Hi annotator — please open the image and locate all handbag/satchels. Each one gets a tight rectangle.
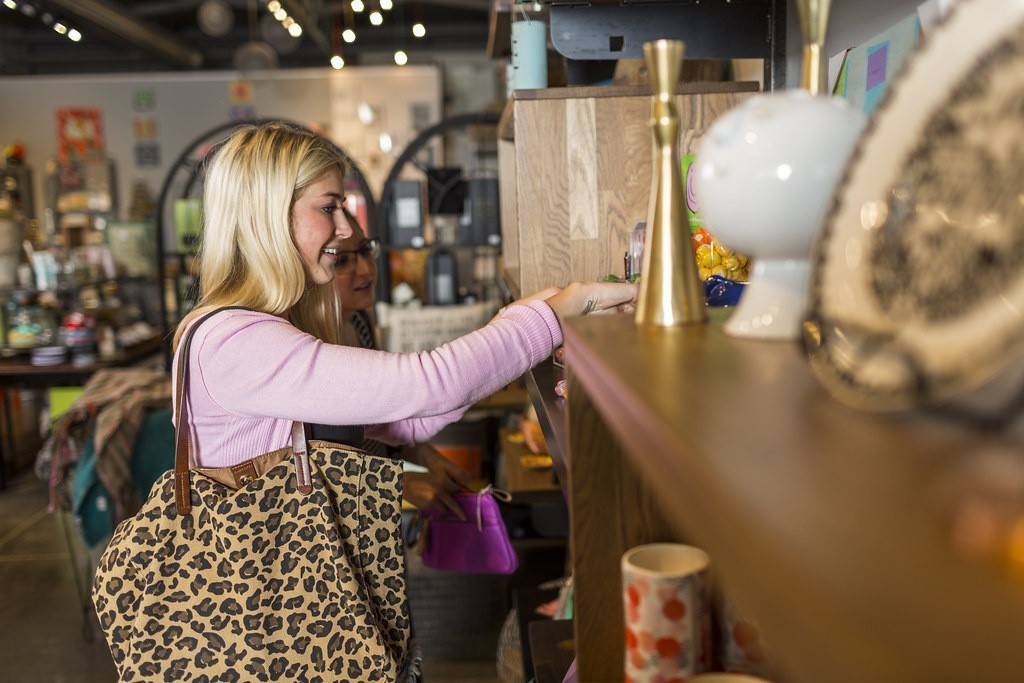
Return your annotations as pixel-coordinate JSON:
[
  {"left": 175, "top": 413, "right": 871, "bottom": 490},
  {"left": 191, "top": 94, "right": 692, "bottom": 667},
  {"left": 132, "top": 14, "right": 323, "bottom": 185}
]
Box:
[
  {"left": 422, "top": 485, "right": 517, "bottom": 574},
  {"left": 92, "top": 305, "right": 413, "bottom": 683}
]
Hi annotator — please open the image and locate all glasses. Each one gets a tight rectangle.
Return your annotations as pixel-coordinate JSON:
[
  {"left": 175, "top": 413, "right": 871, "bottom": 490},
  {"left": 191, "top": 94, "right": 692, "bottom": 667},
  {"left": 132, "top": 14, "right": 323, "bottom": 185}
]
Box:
[{"left": 334, "top": 236, "right": 382, "bottom": 275}]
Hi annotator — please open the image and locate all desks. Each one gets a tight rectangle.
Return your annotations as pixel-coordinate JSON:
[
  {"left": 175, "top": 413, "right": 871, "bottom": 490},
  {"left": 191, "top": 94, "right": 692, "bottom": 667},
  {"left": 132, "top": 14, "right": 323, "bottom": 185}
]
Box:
[{"left": 0, "top": 331, "right": 174, "bottom": 479}]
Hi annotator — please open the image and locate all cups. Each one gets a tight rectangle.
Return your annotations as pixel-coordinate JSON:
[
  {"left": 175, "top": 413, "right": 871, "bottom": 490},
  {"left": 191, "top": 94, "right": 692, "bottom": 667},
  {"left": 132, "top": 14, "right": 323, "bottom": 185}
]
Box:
[{"left": 621, "top": 543, "right": 712, "bottom": 682}]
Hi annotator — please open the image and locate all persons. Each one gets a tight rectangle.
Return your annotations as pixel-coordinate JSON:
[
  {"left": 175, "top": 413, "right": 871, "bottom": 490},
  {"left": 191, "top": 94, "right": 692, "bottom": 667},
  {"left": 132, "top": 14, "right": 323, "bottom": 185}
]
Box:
[{"left": 173, "top": 119, "right": 640, "bottom": 682}]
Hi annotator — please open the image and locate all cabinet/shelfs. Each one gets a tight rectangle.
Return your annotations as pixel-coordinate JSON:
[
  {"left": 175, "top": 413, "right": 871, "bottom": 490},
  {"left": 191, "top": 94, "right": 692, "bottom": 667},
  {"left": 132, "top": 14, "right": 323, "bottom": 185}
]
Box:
[{"left": 496, "top": 81, "right": 1024, "bottom": 683}]
